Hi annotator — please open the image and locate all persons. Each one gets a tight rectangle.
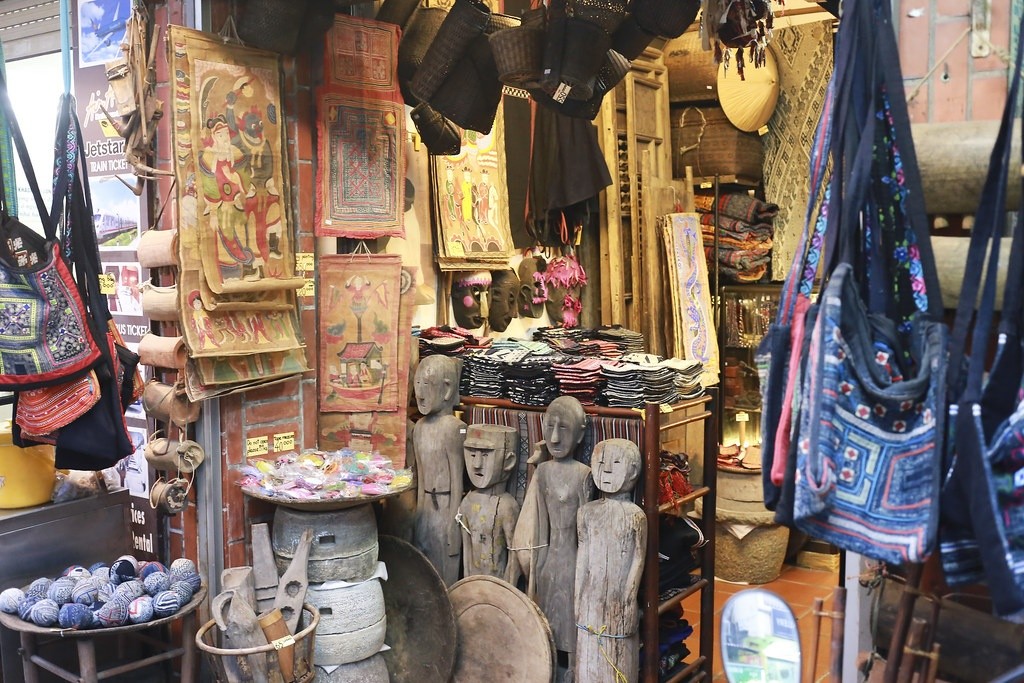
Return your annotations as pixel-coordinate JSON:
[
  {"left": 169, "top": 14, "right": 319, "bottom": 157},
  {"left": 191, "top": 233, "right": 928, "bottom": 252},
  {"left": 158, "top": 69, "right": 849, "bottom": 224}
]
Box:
[
  {"left": 461, "top": 424, "right": 520, "bottom": 585},
  {"left": 413, "top": 353, "right": 467, "bottom": 589},
  {"left": 573, "top": 438, "right": 648, "bottom": 683},
  {"left": 511, "top": 396, "right": 592, "bottom": 683}
]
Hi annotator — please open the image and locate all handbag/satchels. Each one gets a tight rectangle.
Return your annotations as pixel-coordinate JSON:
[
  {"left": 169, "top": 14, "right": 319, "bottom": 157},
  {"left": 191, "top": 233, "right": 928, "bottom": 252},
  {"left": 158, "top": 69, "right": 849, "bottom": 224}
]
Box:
[
  {"left": 751, "top": 1, "right": 1024, "bottom": 614},
  {"left": 0, "top": 74, "right": 139, "bottom": 473}
]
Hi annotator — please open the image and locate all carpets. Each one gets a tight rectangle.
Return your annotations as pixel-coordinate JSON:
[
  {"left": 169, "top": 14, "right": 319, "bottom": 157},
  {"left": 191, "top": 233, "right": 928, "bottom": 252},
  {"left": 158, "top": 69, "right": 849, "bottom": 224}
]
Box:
[{"left": 313, "top": 14, "right": 407, "bottom": 241}]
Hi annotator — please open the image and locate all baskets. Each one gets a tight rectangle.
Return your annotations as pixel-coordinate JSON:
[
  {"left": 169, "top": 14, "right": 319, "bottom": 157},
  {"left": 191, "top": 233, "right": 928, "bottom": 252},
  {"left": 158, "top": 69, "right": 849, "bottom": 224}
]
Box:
[{"left": 373, "top": 0, "right": 699, "bottom": 156}]
